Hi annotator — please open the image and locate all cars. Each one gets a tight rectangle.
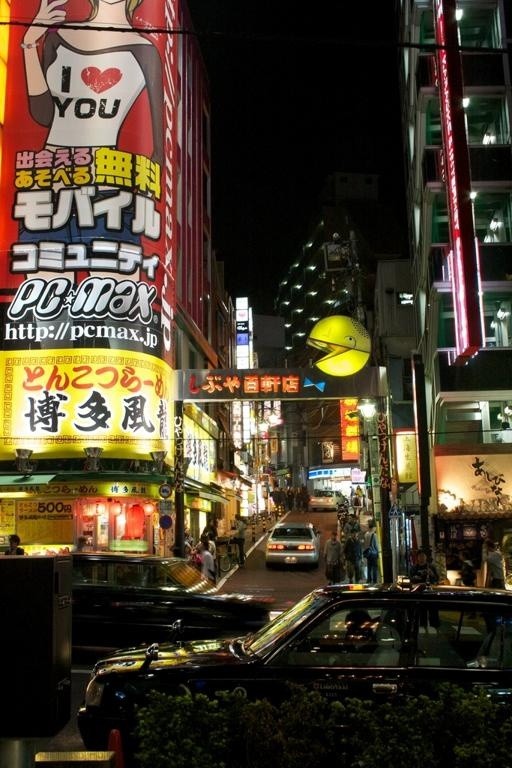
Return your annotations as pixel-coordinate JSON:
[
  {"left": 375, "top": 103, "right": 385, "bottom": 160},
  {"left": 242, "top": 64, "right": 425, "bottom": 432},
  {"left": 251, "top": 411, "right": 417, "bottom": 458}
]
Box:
[{"left": 308, "top": 490, "right": 347, "bottom": 511}]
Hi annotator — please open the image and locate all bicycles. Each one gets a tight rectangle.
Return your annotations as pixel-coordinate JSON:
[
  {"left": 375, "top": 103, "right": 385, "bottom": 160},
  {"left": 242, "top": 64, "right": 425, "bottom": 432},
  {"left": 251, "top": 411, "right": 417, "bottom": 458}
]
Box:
[{"left": 215, "top": 532, "right": 248, "bottom": 572}]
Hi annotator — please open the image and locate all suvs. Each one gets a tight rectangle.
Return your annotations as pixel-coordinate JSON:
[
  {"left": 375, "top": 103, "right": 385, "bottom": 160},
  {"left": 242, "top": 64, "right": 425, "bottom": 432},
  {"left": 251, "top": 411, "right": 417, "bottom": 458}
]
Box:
[
  {"left": 74, "top": 553, "right": 273, "bottom": 666},
  {"left": 76, "top": 583, "right": 511, "bottom": 752}
]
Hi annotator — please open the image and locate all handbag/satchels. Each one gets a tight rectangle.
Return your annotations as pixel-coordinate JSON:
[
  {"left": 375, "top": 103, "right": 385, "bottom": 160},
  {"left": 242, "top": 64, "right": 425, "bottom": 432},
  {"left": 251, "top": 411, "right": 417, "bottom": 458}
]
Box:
[{"left": 362, "top": 546, "right": 379, "bottom": 559}]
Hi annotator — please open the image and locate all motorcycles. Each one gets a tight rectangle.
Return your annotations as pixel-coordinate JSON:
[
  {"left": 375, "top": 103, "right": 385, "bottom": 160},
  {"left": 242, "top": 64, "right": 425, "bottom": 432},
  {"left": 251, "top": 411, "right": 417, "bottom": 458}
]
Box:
[{"left": 337, "top": 500, "right": 349, "bottom": 528}]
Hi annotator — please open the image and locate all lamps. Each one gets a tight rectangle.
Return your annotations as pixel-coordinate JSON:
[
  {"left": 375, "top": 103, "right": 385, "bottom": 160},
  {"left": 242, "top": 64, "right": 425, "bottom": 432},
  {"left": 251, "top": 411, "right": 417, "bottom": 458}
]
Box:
[
  {"left": 84, "top": 447, "right": 104, "bottom": 472},
  {"left": 149, "top": 451, "right": 167, "bottom": 471},
  {"left": 16, "top": 448, "right": 33, "bottom": 472}
]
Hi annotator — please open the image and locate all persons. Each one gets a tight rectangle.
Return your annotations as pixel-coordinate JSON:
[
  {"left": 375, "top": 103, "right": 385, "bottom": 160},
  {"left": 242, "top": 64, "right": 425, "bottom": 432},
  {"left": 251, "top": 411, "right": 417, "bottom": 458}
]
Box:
[
  {"left": 408, "top": 550, "right": 440, "bottom": 629},
  {"left": 362, "top": 522, "right": 379, "bottom": 584},
  {"left": 314, "top": 609, "right": 377, "bottom": 667},
  {"left": 218, "top": 513, "right": 246, "bottom": 567},
  {"left": 196, "top": 540, "right": 216, "bottom": 586},
  {"left": 285, "top": 483, "right": 310, "bottom": 511},
  {"left": 343, "top": 529, "right": 362, "bottom": 583},
  {"left": 322, "top": 530, "right": 343, "bottom": 585},
  {"left": 5, "top": 535, "right": 25, "bottom": 556},
  {"left": 482, "top": 537, "right": 506, "bottom": 590},
  {"left": 182, "top": 518, "right": 218, "bottom": 561}
]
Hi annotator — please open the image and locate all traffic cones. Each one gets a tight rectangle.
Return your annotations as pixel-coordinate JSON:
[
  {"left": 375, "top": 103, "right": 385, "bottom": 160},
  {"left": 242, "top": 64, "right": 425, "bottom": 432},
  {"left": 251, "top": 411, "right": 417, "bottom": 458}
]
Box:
[{"left": 106, "top": 726, "right": 124, "bottom": 766}]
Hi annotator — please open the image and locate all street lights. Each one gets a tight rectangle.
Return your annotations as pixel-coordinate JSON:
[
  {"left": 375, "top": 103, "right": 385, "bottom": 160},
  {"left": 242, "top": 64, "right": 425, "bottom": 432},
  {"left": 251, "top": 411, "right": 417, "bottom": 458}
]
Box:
[{"left": 355, "top": 396, "right": 393, "bottom": 586}]
